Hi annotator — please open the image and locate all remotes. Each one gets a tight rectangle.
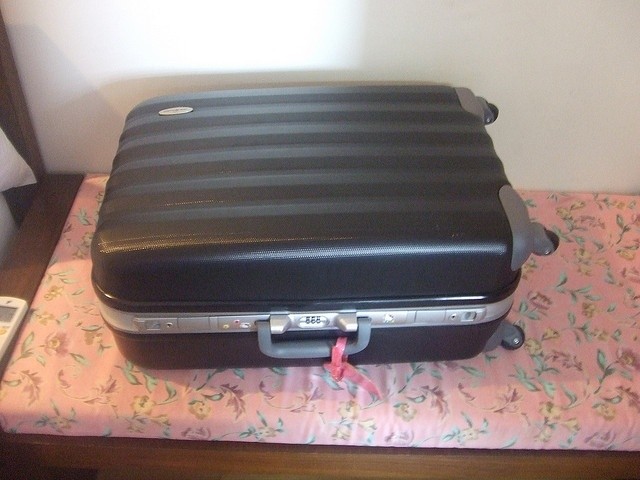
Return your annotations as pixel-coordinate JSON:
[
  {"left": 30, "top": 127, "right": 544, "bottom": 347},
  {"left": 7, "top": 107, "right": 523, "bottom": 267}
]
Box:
[{"left": 0, "top": 296, "right": 28, "bottom": 361}]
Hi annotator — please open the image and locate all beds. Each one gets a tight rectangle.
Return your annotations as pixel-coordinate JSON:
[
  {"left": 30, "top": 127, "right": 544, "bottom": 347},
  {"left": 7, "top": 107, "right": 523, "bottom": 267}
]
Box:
[{"left": 1, "top": 7, "right": 639, "bottom": 480}]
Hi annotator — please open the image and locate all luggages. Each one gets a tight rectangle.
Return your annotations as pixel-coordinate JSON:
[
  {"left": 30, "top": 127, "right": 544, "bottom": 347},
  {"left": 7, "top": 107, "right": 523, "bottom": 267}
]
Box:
[{"left": 90, "top": 85, "right": 558, "bottom": 369}]
